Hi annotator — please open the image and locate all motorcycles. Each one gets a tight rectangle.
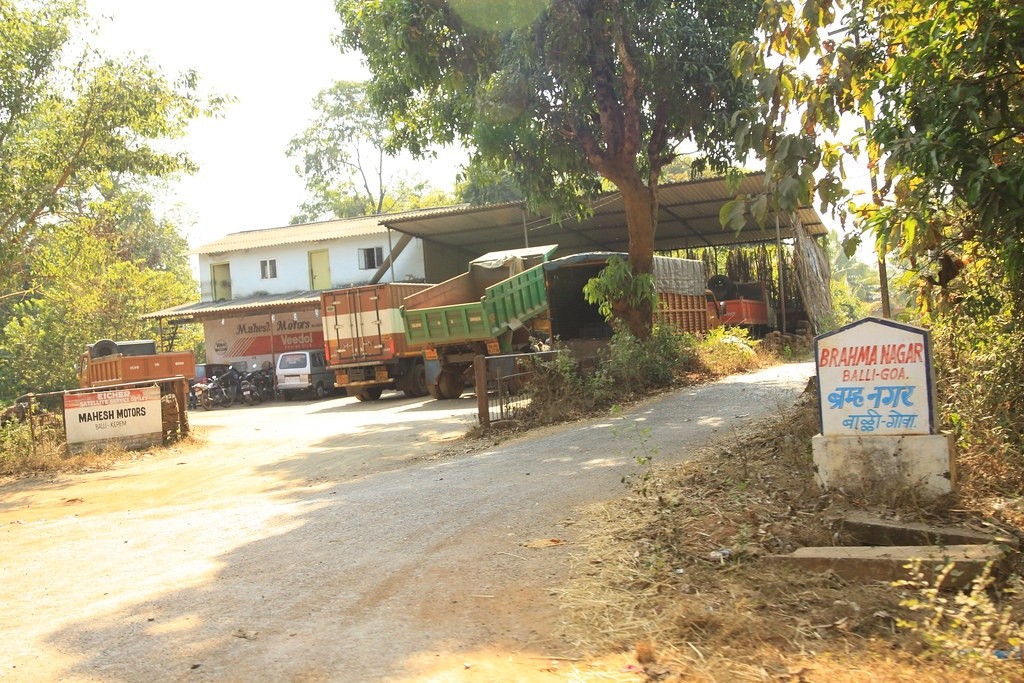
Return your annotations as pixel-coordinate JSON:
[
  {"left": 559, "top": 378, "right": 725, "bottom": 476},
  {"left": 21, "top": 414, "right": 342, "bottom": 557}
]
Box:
[{"left": 189, "top": 363, "right": 277, "bottom": 411}]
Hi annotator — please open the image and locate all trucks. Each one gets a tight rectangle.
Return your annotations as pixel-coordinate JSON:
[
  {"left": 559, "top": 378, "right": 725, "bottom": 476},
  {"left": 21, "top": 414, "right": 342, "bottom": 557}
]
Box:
[
  {"left": 398, "top": 243, "right": 559, "bottom": 401},
  {"left": 319, "top": 282, "right": 439, "bottom": 402},
  {"left": 77, "top": 339, "right": 195, "bottom": 404},
  {"left": 541, "top": 252, "right": 777, "bottom": 359}
]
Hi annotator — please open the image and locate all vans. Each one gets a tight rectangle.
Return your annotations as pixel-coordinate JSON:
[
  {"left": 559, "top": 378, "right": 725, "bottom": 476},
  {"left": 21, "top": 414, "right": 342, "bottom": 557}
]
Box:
[{"left": 273, "top": 349, "right": 336, "bottom": 399}]
